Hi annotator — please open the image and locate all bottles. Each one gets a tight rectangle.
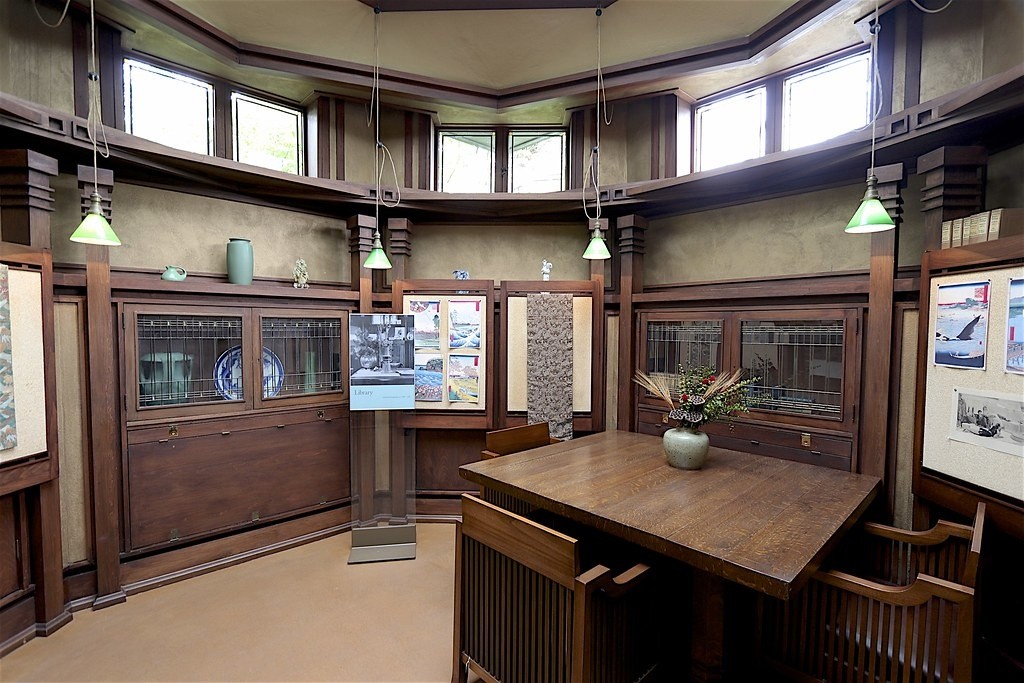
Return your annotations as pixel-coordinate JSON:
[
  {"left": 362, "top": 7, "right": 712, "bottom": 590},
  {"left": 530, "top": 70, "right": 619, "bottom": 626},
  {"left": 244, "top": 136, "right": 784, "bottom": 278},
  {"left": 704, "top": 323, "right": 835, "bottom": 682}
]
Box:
[{"left": 227, "top": 237, "right": 253, "bottom": 285}]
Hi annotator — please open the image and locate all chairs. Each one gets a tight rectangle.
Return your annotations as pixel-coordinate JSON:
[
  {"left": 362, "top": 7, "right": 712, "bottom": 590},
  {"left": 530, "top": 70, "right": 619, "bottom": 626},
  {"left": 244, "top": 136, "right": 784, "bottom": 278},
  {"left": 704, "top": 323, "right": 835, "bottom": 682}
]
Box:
[
  {"left": 452, "top": 489, "right": 700, "bottom": 683},
  {"left": 480, "top": 422, "right": 564, "bottom": 521},
  {"left": 786, "top": 499, "right": 986, "bottom": 683}
]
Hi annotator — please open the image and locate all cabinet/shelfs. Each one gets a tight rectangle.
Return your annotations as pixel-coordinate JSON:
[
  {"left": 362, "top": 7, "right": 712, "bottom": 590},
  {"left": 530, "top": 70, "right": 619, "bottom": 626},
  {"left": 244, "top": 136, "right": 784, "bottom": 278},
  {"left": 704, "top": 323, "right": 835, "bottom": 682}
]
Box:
[
  {"left": 116, "top": 297, "right": 349, "bottom": 554},
  {"left": 635, "top": 308, "right": 866, "bottom": 473}
]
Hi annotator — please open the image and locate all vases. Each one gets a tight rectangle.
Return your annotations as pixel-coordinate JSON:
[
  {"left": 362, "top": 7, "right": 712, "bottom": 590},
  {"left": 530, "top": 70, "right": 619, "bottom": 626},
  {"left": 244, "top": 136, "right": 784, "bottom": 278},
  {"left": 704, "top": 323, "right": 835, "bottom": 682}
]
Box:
[
  {"left": 663, "top": 427, "right": 709, "bottom": 469},
  {"left": 227, "top": 236, "right": 253, "bottom": 284}
]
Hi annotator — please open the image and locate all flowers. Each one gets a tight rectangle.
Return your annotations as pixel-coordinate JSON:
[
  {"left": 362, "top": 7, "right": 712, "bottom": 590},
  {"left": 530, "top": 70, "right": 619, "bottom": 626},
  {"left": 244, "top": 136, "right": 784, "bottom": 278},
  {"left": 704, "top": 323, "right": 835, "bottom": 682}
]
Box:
[{"left": 634, "top": 362, "right": 768, "bottom": 429}]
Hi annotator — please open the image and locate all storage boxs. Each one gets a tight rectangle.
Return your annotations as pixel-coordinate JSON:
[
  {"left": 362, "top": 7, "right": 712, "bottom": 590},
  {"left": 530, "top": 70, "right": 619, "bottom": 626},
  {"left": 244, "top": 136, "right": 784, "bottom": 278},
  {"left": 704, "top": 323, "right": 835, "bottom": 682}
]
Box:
[{"left": 940, "top": 206, "right": 1024, "bottom": 249}]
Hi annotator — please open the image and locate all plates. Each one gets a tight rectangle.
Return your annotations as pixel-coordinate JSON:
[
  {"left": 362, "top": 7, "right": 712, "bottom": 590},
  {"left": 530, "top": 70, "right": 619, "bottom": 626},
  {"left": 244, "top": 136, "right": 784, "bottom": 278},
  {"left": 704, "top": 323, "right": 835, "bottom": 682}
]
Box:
[{"left": 212, "top": 344, "right": 284, "bottom": 401}]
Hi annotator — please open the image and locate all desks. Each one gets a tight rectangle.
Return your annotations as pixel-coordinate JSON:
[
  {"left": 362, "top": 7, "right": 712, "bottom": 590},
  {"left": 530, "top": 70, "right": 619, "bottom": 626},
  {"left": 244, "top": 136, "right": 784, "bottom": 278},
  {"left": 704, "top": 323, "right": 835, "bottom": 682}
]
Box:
[{"left": 458, "top": 430, "right": 881, "bottom": 683}]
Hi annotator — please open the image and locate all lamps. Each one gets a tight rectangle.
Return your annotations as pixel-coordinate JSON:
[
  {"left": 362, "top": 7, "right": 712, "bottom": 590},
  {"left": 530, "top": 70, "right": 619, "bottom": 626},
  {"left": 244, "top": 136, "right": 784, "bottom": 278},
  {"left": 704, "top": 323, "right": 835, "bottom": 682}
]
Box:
[
  {"left": 69, "top": 0, "right": 121, "bottom": 246},
  {"left": 845, "top": 0, "right": 895, "bottom": 236},
  {"left": 582, "top": 0, "right": 612, "bottom": 260},
  {"left": 363, "top": 6, "right": 393, "bottom": 270}
]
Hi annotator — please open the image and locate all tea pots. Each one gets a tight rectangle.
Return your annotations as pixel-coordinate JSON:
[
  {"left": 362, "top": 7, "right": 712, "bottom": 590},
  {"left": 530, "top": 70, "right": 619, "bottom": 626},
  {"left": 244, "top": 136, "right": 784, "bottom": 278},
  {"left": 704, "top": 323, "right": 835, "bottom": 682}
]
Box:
[{"left": 161, "top": 265, "right": 186, "bottom": 281}]
